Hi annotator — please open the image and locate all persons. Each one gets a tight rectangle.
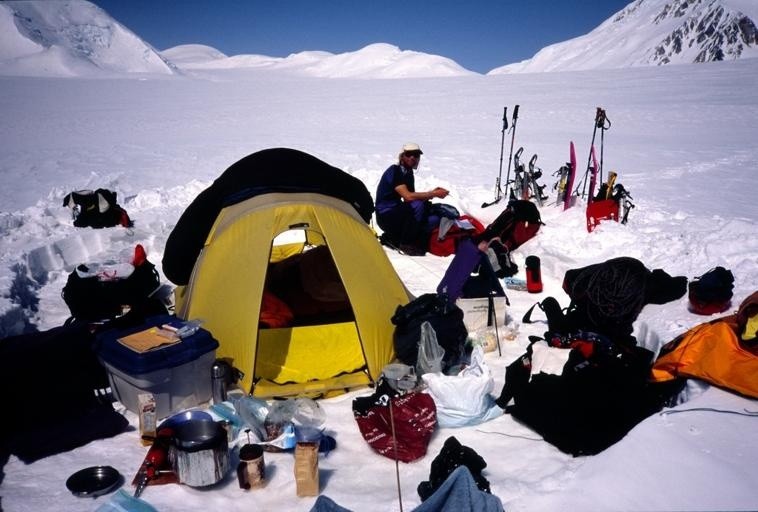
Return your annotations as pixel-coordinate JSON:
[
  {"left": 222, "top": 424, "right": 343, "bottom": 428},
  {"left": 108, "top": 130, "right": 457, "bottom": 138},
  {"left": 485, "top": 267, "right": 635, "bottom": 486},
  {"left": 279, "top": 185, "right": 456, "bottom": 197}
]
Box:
[{"left": 374, "top": 142, "right": 451, "bottom": 257}]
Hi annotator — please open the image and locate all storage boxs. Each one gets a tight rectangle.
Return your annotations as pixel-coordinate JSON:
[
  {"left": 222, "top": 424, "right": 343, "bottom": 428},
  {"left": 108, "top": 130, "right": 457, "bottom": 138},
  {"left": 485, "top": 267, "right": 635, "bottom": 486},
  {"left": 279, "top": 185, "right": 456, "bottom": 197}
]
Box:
[
  {"left": 95, "top": 313, "right": 219, "bottom": 422},
  {"left": 455, "top": 296, "right": 506, "bottom": 333}
]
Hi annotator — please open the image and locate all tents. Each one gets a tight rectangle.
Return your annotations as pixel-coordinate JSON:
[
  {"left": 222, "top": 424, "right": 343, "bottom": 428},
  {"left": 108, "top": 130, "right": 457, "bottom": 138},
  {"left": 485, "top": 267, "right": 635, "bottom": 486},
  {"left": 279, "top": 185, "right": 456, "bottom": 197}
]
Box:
[{"left": 161, "top": 146, "right": 419, "bottom": 403}]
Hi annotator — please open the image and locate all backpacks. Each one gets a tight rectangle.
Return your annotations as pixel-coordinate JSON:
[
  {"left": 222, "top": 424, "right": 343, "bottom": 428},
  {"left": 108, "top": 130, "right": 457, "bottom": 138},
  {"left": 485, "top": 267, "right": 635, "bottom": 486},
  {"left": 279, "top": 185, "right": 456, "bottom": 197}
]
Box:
[
  {"left": 494, "top": 337, "right": 686, "bottom": 457},
  {"left": 478, "top": 200, "right": 546, "bottom": 249}
]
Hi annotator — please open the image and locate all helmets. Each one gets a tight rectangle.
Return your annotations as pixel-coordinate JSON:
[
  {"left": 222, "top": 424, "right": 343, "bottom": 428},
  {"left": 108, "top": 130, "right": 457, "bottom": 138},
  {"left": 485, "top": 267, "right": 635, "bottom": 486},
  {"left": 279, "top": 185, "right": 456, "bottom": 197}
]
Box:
[{"left": 690, "top": 266, "right": 735, "bottom": 316}]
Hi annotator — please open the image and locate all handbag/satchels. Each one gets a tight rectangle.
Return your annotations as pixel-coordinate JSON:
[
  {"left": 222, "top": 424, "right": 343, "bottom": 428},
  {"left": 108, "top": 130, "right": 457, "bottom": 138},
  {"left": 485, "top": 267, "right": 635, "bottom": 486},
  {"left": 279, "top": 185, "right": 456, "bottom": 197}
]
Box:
[
  {"left": 352, "top": 377, "right": 436, "bottom": 463},
  {"left": 430, "top": 215, "right": 485, "bottom": 257}
]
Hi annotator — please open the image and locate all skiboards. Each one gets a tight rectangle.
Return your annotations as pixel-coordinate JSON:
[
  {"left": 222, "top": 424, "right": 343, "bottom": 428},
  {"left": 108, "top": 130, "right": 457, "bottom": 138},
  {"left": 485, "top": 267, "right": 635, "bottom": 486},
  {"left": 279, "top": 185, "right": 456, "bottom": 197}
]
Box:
[{"left": 481, "top": 142, "right": 628, "bottom": 225}]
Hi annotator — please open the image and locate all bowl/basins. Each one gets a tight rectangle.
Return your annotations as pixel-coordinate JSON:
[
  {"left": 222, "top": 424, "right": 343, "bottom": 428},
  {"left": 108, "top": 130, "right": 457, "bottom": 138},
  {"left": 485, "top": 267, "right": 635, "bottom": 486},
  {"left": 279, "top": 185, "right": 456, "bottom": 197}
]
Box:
[
  {"left": 160, "top": 409, "right": 212, "bottom": 423},
  {"left": 64, "top": 465, "right": 121, "bottom": 498}
]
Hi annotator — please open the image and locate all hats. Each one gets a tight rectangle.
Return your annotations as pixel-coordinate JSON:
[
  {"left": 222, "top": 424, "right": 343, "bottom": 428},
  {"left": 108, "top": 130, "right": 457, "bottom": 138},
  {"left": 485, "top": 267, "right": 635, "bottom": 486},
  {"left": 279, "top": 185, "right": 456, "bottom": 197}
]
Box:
[{"left": 403, "top": 143, "right": 423, "bottom": 155}]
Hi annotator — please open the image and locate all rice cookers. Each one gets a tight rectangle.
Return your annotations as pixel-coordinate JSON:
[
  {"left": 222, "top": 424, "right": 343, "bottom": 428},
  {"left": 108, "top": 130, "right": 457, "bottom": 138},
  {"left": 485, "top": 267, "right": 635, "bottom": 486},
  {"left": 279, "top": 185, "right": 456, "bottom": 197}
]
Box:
[{"left": 169, "top": 418, "right": 230, "bottom": 489}]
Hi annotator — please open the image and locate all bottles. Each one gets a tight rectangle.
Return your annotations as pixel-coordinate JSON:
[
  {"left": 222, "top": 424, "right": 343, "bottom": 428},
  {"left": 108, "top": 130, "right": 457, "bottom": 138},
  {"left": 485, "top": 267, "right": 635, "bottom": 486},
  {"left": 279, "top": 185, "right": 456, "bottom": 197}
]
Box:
[
  {"left": 235, "top": 441, "right": 266, "bottom": 491},
  {"left": 524, "top": 255, "right": 542, "bottom": 293},
  {"left": 211, "top": 363, "right": 228, "bottom": 404}
]
